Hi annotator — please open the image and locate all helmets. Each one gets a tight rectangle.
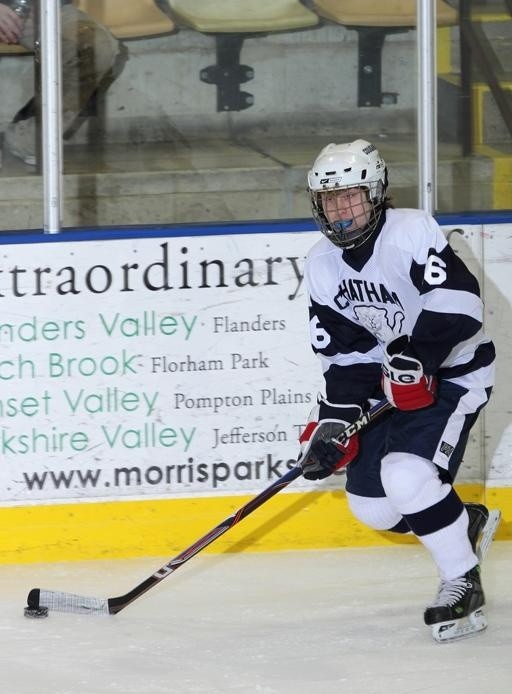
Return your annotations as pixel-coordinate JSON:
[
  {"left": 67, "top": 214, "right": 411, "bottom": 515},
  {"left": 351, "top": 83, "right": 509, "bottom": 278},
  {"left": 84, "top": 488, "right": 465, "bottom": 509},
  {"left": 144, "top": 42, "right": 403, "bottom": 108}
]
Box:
[{"left": 306, "top": 139, "right": 389, "bottom": 208}]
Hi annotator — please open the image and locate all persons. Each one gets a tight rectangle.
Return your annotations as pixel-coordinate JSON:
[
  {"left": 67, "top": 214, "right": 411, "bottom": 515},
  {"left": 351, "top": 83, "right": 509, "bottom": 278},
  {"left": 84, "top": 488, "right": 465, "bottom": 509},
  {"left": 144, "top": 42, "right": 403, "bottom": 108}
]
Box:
[
  {"left": 294, "top": 136, "right": 499, "bottom": 626},
  {"left": 0, "top": 0, "right": 120, "bottom": 168}
]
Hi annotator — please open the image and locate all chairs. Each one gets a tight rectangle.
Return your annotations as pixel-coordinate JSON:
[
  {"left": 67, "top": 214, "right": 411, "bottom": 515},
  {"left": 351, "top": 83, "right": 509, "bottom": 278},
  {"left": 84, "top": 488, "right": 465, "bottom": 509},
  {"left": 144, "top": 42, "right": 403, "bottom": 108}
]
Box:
[
  {"left": 3, "top": 1, "right": 46, "bottom": 65},
  {"left": 166, "top": 0, "right": 323, "bottom": 110},
  {"left": 68, "top": 0, "right": 178, "bottom": 44},
  {"left": 309, "top": 0, "right": 459, "bottom": 108}
]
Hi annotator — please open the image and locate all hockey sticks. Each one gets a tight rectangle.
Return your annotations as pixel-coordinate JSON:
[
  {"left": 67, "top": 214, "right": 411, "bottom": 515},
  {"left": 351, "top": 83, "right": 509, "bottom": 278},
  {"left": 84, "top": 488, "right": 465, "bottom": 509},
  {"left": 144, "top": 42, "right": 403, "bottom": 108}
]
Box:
[{"left": 28, "top": 398, "right": 395, "bottom": 616}]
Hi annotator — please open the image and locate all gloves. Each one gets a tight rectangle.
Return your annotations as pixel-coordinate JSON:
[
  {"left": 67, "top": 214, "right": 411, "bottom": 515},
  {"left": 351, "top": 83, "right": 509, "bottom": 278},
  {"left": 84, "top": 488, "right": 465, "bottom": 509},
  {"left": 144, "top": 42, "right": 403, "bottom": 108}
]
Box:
[
  {"left": 379, "top": 334, "right": 439, "bottom": 412},
  {"left": 298, "top": 393, "right": 368, "bottom": 483}
]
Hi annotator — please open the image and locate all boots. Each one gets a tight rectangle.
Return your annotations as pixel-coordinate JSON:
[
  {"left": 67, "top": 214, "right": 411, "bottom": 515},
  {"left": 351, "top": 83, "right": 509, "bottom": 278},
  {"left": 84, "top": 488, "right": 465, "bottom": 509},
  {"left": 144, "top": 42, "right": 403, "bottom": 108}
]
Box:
[
  {"left": 466, "top": 501, "right": 488, "bottom": 553},
  {"left": 422, "top": 568, "right": 486, "bottom": 624}
]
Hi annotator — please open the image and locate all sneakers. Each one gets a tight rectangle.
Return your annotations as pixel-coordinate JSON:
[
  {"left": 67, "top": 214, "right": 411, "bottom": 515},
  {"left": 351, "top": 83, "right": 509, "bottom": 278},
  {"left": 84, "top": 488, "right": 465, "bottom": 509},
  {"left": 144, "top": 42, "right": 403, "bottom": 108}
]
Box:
[{"left": 6, "top": 134, "right": 38, "bottom": 166}]
